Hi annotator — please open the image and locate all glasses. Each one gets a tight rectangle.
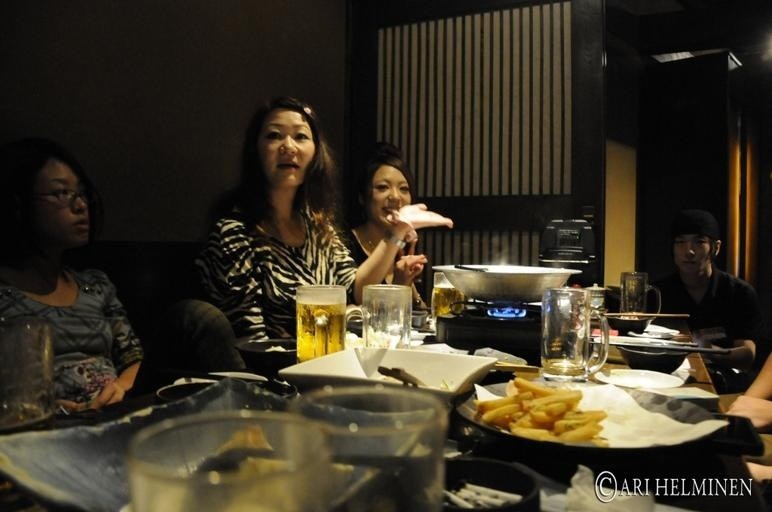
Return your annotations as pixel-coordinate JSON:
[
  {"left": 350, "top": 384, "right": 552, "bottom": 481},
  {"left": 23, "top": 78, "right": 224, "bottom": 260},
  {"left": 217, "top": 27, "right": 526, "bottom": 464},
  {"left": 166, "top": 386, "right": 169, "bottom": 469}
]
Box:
[{"left": 37, "top": 188, "right": 95, "bottom": 209}]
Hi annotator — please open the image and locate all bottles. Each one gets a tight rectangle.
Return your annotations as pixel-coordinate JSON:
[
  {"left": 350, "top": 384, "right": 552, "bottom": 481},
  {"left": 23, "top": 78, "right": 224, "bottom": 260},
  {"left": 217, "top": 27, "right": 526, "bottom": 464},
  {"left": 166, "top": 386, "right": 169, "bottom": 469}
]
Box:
[{"left": 586, "top": 283, "right": 607, "bottom": 310}]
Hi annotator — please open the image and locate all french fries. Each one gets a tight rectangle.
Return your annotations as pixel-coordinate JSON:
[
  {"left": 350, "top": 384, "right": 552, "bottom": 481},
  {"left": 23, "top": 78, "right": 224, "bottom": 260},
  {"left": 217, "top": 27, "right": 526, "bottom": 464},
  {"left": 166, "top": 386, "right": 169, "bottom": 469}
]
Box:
[{"left": 473, "top": 377, "right": 609, "bottom": 448}]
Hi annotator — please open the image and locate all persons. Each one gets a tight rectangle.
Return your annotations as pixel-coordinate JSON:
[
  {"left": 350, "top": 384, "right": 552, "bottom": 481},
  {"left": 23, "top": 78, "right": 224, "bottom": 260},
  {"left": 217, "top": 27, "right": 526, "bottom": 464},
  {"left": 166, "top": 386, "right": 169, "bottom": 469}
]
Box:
[
  {"left": 197, "top": 92, "right": 453, "bottom": 347},
  {"left": 334, "top": 139, "right": 431, "bottom": 312},
  {"left": 641, "top": 210, "right": 772, "bottom": 371},
  {"left": 726, "top": 350, "right": 772, "bottom": 485},
  {"left": 0, "top": 134, "right": 143, "bottom": 512}
]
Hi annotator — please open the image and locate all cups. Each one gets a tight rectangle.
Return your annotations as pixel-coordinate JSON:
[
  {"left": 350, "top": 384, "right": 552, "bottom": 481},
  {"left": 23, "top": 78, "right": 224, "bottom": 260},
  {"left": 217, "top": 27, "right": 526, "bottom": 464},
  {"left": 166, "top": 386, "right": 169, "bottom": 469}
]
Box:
[
  {"left": 295, "top": 285, "right": 346, "bottom": 363},
  {"left": 0, "top": 318, "right": 57, "bottom": 429},
  {"left": 346, "top": 283, "right": 413, "bottom": 347},
  {"left": 296, "top": 385, "right": 447, "bottom": 512},
  {"left": 540, "top": 287, "right": 610, "bottom": 380},
  {"left": 127, "top": 412, "right": 335, "bottom": 512},
  {"left": 430, "top": 271, "right": 465, "bottom": 323}
]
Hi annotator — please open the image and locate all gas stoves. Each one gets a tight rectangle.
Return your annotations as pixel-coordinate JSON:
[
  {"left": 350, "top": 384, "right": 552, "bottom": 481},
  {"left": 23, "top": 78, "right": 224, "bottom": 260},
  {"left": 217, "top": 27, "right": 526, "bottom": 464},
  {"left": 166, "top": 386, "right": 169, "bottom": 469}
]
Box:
[{"left": 434, "top": 300, "right": 541, "bottom": 360}]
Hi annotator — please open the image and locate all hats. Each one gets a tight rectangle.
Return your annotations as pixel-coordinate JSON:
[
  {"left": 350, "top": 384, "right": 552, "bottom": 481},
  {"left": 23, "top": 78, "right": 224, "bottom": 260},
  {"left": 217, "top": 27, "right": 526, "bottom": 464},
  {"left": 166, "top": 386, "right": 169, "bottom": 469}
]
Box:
[{"left": 666, "top": 209, "right": 719, "bottom": 244}]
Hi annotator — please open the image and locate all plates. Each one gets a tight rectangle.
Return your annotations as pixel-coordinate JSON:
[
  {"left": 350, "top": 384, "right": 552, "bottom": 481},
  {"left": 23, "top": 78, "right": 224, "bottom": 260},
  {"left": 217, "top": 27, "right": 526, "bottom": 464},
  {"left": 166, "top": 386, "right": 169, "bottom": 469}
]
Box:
[
  {"left": 156, "top": 381, "right": 295, "bottom": 402},
  {"left": 276, "top": 342, "right": 500, "bottom": 397},
  {"left": 0, "top": 378, "right": 436, "bottom": 512},
  {"left": 596, "top": 306, "right": 694, "bottom": 393}
]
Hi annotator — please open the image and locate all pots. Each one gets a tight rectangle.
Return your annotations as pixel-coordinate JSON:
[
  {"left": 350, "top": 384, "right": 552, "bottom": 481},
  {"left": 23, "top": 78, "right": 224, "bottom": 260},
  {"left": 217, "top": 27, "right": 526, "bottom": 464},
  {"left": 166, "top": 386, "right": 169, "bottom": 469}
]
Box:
[{"left": 451, "top": 380, "right": 764, "bottom": 490}]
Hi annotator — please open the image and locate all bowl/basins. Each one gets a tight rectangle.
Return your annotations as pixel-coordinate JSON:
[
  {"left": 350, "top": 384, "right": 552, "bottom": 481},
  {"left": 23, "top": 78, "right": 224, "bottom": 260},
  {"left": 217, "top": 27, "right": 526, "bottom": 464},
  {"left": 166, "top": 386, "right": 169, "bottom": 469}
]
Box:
[
  {"left": 231, "top": 338, "right": 296, "bottom": 376},
  {"left": 429, "top": 264, "right": 582, "bottom": 300},
  {"left": 330, "top": 452, "right": 541, "bottom": 511}
]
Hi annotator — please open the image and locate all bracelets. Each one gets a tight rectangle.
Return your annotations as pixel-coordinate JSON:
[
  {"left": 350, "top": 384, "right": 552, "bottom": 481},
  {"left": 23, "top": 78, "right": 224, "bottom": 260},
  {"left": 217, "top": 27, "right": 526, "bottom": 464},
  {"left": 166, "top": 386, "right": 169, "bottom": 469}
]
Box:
[
  {"left": 382, "top": 229, "right": 407, "bottom": 249},
  {"left": 412, "top": 293, "right": 422, "bottom": 310}
]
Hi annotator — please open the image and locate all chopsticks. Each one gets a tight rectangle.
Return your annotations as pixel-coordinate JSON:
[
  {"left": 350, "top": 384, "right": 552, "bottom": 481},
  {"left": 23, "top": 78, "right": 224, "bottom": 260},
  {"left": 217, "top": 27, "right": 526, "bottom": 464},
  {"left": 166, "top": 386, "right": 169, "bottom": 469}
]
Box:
[
  {"left": 588, "top": 339, "right": 732, "bottom": 356},
  {"left": 378, "top": 364, "right": 425, "bottom": 390},
  {"left": 455, "top": 265, "right": 488, "bottom": 272}
]
